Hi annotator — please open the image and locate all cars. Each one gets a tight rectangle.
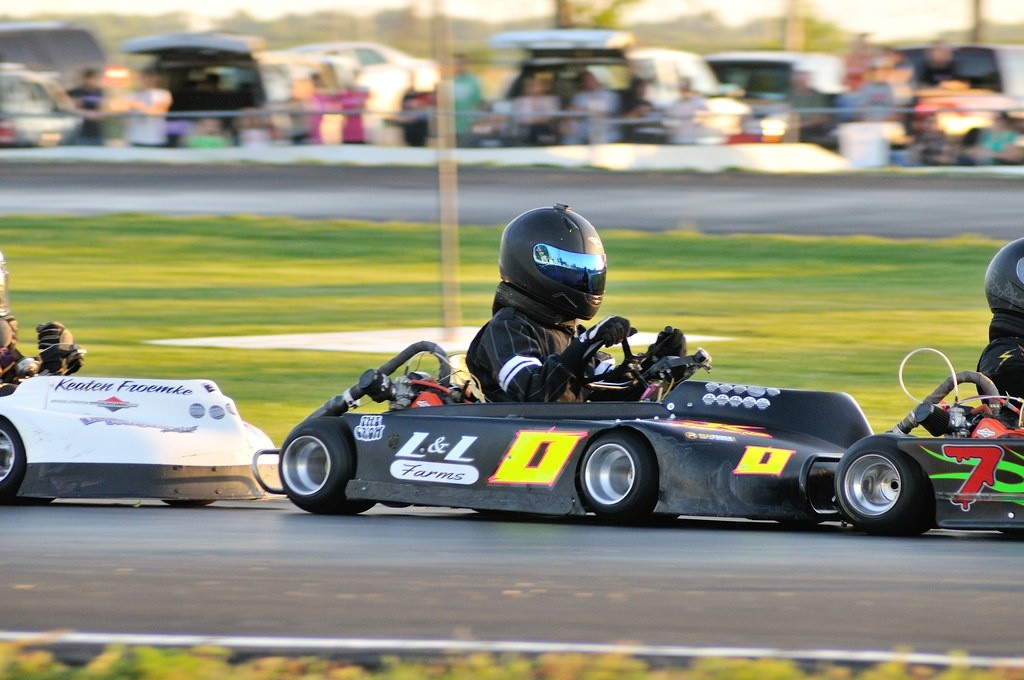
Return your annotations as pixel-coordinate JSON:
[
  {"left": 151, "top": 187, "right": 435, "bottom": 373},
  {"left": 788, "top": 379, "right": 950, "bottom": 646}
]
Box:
[{"left": 0, "top": 26, "right": 1023, "bottom": 149}]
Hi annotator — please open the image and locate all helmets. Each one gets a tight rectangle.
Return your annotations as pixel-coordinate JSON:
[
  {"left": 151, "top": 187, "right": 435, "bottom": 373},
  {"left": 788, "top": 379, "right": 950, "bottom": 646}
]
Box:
[
  {"left": 985, "top": 238, "right": 1024, "bottom": 313},
  {"left": 499, "top": 202, "right": 606, "bottom": 320}
]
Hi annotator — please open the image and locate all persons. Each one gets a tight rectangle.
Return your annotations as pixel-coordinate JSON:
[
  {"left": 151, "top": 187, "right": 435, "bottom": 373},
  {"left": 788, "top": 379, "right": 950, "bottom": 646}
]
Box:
[
  {"left": 0, "top": 250, "right": 83, "bottom": 384},
  {"left": 974, "top": 234, "right": 1024, "bottom": 428},
  {"left": 467, "top": 205, "right": 688, "bottom": 403},
  {"left": 54, "top": 32, "right": 1024, "bottom": 167}
]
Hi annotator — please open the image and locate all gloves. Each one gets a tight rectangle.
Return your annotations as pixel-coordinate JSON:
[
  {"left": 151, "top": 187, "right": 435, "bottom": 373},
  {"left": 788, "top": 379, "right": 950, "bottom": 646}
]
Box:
[
  {"left": 560, "top": 315, "right": 638, "bottom": 375},
  {"left": 655, "top": 326, "right": 687, "bottom": 360},
  {"left": 36, "top": 321, "right": 74, "bottom": 350}
]
